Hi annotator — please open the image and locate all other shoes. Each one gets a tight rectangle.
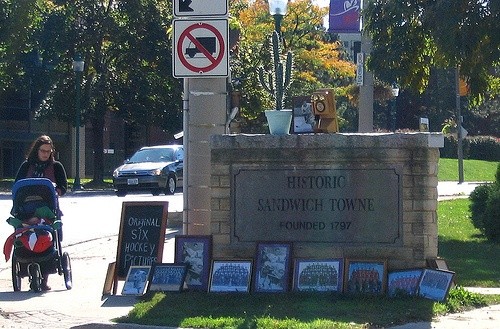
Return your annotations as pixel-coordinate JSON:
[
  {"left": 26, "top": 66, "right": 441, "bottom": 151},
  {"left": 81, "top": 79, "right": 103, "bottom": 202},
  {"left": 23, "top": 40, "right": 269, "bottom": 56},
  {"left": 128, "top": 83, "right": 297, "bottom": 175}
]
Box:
[{"left": 41, "top": 274, "right": 52, "bottom": 290}]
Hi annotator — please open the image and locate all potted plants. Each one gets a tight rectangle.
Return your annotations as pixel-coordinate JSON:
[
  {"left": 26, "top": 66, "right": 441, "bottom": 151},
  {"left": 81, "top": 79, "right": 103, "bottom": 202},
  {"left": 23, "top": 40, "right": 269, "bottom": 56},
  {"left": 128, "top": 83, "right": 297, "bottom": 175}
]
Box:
[{"left": 258, "top": 29, "right": 294, "bottom": 133}]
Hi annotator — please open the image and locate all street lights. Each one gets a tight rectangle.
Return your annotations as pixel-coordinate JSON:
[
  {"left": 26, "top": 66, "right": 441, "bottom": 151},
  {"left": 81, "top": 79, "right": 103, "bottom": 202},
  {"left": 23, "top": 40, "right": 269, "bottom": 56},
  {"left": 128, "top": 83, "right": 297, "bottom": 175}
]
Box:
[
  {"left": 267, "top": 0, "right": 288, "bottom": 35},
  {"left": 70, "top": 50, "right": 85, "bottom": 192}
]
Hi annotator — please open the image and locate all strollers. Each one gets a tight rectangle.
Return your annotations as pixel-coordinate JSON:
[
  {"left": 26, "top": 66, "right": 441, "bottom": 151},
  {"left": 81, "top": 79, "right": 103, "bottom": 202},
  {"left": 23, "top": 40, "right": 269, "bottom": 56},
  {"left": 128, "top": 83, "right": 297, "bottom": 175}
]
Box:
[{"left": 12, "top": 178, "right": 72, "bottom": 292}]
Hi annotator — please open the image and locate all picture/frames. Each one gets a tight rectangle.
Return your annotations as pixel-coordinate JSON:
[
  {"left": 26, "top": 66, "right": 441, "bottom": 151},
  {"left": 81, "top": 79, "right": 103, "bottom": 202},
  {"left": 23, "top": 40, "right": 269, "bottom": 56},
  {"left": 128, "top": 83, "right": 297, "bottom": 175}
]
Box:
[
  {"left": 292, "top": 256, "right": 346, "bottom": 292},
  {"left": 251, "top": 241, "right": 294, "bottom": 293},
  {"left": 149, "top": 263, "right": 188, "bottom": 293},
  {"left": 208, "top": 258, "right": 255, "bottom": 293},
  {"left": 344, "top": 258, "right": 388, "bottom": 296},
  {"left": 121, "top": 265, "right": 152, "bottom": 295},
  {"left": 174, "top": 234, "right": 214, "bottom": 290},
  {"left": 385, "top": 268, "right": 424, "bottom": 295},
  {"left": 415, "top": 269, "right": 455, "bottom": 302}
]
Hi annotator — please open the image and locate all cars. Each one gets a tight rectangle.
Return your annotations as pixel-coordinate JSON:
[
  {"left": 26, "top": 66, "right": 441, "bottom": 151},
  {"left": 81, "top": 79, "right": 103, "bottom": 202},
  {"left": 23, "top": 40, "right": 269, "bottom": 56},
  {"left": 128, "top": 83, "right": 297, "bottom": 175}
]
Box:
[{"left": 112, "top": 145, "right": 184, "bottom": 197}]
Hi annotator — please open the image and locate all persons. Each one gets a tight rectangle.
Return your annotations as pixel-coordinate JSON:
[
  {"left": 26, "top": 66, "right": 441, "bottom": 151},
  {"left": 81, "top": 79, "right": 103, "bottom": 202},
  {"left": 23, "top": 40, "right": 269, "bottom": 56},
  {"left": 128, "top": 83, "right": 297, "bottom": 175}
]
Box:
[
  {"left": 152, "top": 267, "right": 183, "bottom": 284},
  {"left": 299, "top": 262, "right": 338, "bottom": 286},
  {"left": 213, "top": 263, "right": 249, "bottom": 287},
  {"left": 134, "top": 269, "right": 143, "bottom": 294},
  {"left": 262, "top": 265, "right": 281, "bottom": 286},
  {"left": 28, "top": 217, "right": 40, "bottom": 225},
  {"left": 351, "top": 266, "right": 379, "bottom": 291},
  {"left": 14, "top": 136, "right": 68, "bottom": 290},
  {"left": 391, "top": 274, "right": 418, "bottom": 291},
  {"left": 192, "top": 244, "right": 199, "bottom": 258}
]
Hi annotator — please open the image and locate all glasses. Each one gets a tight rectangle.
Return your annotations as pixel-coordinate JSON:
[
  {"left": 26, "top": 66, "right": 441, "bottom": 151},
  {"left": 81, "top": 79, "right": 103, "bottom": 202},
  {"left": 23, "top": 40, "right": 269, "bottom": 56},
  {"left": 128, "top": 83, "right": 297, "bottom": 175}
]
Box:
[{"left": 39, "top": 148, "right": 53, "bottom": 153}]
